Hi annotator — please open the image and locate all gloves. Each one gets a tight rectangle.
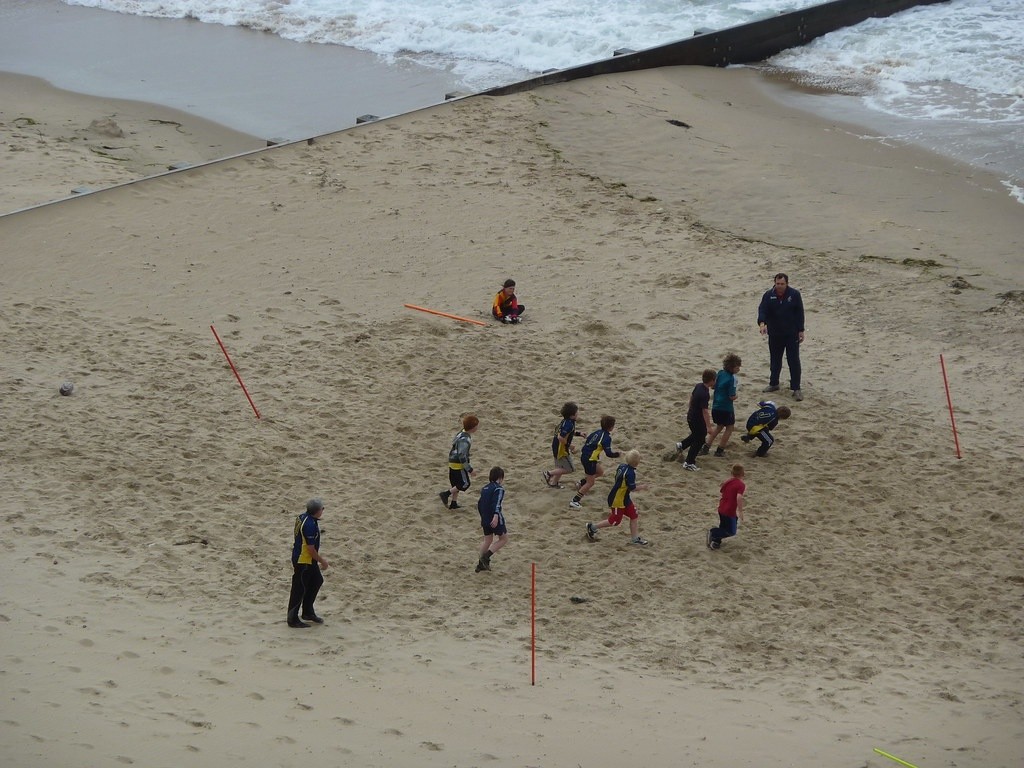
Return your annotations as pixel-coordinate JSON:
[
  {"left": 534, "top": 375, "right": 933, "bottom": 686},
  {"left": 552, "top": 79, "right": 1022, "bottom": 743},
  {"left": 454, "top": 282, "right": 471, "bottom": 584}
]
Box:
[
  {"left": 500, "top": 318, "right": 508, "bottom": 324},
  {"left": 512, "top": 317, "right": 517, "bottom": 323}
]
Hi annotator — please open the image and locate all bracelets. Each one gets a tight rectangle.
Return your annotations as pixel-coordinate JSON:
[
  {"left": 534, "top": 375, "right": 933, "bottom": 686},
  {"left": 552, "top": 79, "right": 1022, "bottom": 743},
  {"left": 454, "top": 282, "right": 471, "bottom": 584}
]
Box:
[{"left": 760, "top": 324, "right": 764, "bottom": 327}]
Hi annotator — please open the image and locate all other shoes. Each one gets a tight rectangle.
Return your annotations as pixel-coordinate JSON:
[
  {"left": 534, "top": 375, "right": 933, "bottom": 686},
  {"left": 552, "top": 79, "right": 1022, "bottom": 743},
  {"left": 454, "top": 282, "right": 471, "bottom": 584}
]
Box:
[
  {"left": 516, "top": 317, "right": 523, "bottom": 323},
  {"left": 449, "top": 505, "right": 461, "bottom": 509},
  {"left": 697, "top": 448, "right": 708, "bottom": 456},
  {"left": 550, "top": 481, "right": 564, "bottom": 489},
  {"left": 794, "top": 389, "right": 803, "bottom": 401},
  {"left": 575, "top": 481, "right": 582, "bottom": 491},
  {"left": 714, "top": 452, "right": 724, "bottom": 457},
  {"left": 505, "top": 315, "right": 512, "bottom": 322},
  {"left": 570, "top": 499, "right": 583, "bottom": 509},
  {"left": 741, "top": 436, "right": 749, "bottom": 444},
  {"left": 543, "top": 471, "right": 551, "bottom": 485},
  {"left": 439, "top": 491, "right": 448, "bottom": 507},
  {"left": 708, "top": 529, "right": 720, "bottom": 550},
  {"left": 756, "top": 451, "right": 768, "bottom": 457},
  {"left": 476, "top": 565, "right": 488, "bottom": 573},
  {"left": 479, "top": 556, "right": 491, "bottom": 571},
  {"left": 763, "top": 384, "right": 780, "bottom": 392}
]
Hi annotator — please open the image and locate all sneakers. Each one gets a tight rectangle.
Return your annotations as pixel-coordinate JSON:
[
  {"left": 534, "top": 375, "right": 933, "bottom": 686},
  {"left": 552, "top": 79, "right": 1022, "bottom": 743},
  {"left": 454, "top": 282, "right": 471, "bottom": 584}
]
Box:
[
  {"left": 683, "top": 461, "right": 700, "bottom": 471},
  {"left": 677, "top": 442, "right": 684, "bottom": 457},
  {"left": 630, "top": 538, "right": 648, "bottom": 545},
  {"left": 585, "top": 522, "right": 596, "bottom": 541},
  {"left": 288, "top": 620, "right": 310, "bottom": 628},
  {"left": 302, "top": 612, "right": 323, "bottom": 623}
]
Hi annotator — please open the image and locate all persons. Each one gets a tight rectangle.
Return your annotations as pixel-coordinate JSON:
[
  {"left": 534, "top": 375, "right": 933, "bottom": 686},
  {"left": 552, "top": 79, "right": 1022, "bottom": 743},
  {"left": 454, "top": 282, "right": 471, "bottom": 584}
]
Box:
[
  {"left": 543, "top": 402, "right": 589, "bottom": 490},
  {"left": 757, "top": 273, "right": 805, "bottom": 401},
  {"left": 439, "top": 416, "right": 479, "bottom": 510},
  {"left": 708, "top": 464, "right": 745, "bottom": 550},
  {"left": 570, "top": 416, "right": 626, "bottom": 510},
  {"left": 491, "top": 279, "right": 525, "bottom": 324},
  {"left": 476, "top": 467, "right": 509, "bottom": 573},
  {"left": 701, "top": 353, "right": 743, "bottom": 457},
  {"left": 286, "top": 499, "right": 328, "bottom": 628},
  {"left": 585, "top": 450, "right": 650, "bottom": 545},
  {"left": 740, "top": 400, "right": 792, "bottom": 457},
  {"left": 674, "top": 369, "right": 717, "bottom": 471}
]
